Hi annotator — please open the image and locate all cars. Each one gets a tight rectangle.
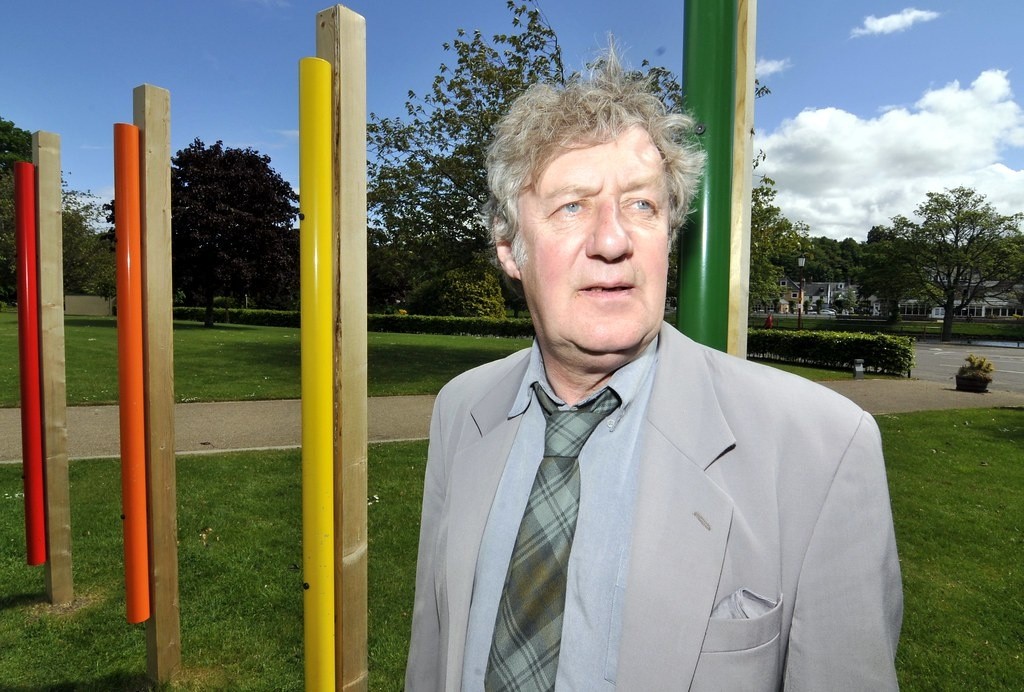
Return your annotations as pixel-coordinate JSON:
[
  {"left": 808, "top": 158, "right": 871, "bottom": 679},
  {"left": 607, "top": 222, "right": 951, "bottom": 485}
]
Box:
[{"left": 807, "top": 307, "right": 873, "bottom": 317}]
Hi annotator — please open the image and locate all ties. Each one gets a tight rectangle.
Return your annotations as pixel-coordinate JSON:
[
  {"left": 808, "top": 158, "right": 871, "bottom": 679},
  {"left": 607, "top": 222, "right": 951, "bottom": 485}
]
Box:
[{"left": 484, "top": 382, "right": 621, "bottom": 692}]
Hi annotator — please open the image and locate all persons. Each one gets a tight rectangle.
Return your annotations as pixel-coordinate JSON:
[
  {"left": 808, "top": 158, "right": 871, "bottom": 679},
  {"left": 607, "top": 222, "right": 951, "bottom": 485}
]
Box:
[
  {"left": 404, "top": 65, "right": 904, "bottom": 692},
  {"left": 765, "top": 313, "right": 772, "bottom": 329}
]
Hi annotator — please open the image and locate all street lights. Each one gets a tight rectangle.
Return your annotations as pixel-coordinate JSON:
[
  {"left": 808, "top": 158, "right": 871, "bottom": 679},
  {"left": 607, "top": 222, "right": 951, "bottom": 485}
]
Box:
[{"left": 797, "top": 253, "right": 807, "bottom": 331}]
